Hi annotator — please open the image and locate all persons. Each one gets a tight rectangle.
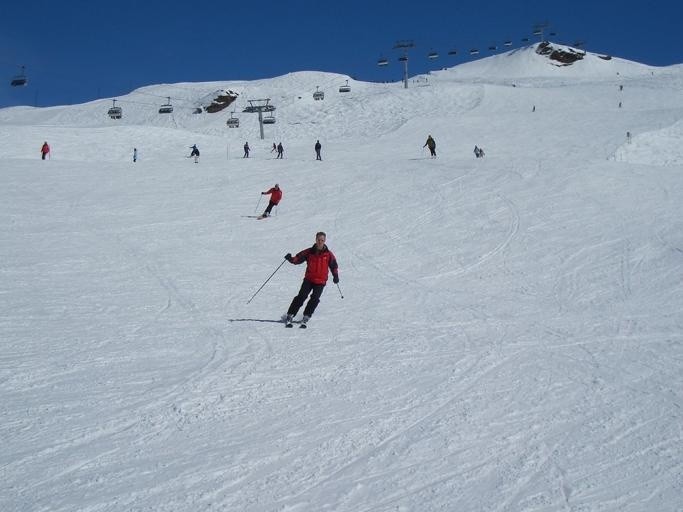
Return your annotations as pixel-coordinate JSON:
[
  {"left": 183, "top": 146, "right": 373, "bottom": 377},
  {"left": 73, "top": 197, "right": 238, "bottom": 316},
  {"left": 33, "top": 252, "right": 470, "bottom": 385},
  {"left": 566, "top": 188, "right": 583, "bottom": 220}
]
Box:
[
  {"left": 314, "top": 139, "right": 321, "bottom": 161},
  {"left": 423, "top": 135, "right": 437, "bottom": 157},
  {"left": 271, "top": 142, "right": 283, "bottom": 159},
  {"left": 243, "top": 141, "right": 250, "bottom": 158},
  {"left": 41, "top": 141, "right": 49, "bottom": 160},
  {"left": 474, "top": 144, "right": 485, "bottom": 159},
  {"left": 134, "top": 148, "right": 138, "bottom": 162},
  {"left": 189, "top": 144, "right": 200, "bottom": 164},
  {"left": 285, "top": 232, "right": 340, "bottom": 328},
  {"left": 261, "top": 183, "right": 283, "bottom": 217}
]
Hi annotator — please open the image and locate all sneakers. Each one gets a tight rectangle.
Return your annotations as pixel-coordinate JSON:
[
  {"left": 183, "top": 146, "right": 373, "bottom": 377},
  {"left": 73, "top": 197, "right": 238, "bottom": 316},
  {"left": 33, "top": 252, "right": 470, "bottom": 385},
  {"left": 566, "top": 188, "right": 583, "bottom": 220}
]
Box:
[
  {"left": 301, "top": 316, "right": 308, "bottom": 324},
  {"left": 287, "top": 314, "right": 293, "bottom": 322}
]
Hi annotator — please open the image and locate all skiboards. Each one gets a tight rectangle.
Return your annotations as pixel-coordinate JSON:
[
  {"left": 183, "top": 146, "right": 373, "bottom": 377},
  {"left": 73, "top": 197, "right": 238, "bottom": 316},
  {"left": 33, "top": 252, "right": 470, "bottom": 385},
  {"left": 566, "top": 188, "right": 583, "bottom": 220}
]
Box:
[{"left": 286, "top": 320, "right": 308, "bottom": 328}]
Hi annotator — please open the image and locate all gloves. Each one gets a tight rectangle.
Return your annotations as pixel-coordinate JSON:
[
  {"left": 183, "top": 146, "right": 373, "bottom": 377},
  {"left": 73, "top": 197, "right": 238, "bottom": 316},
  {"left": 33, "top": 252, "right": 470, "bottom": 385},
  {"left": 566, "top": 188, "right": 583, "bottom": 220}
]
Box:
[
  {"left": 285, "top": 254, "right": 291, "bottom": 259},
  {"left": 333, "top": 277, "right": 339, "bottom": 283}
]
